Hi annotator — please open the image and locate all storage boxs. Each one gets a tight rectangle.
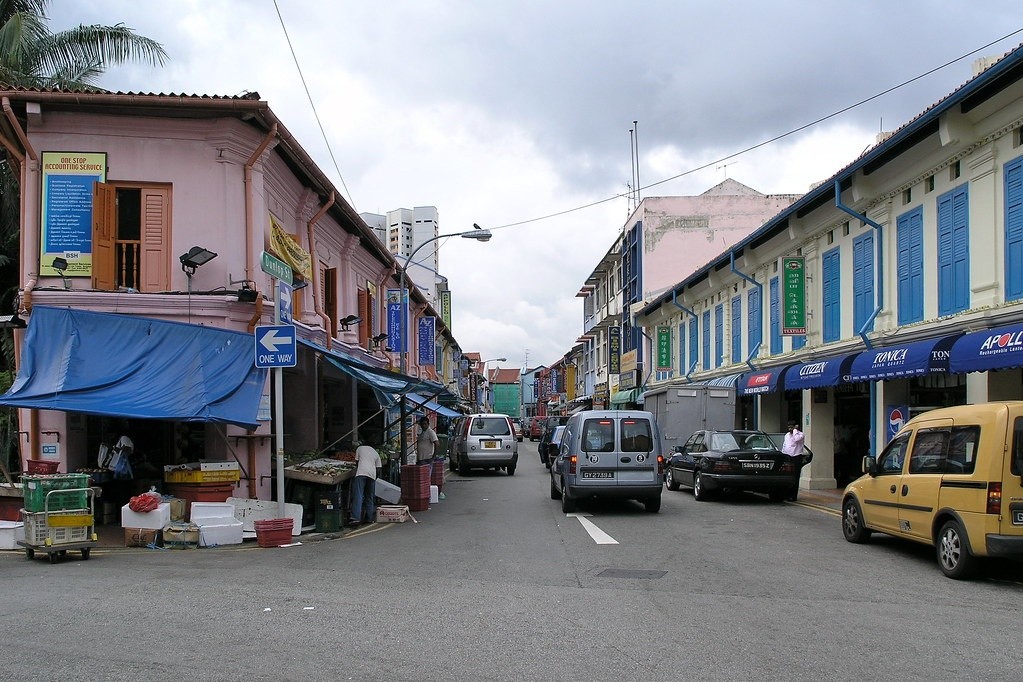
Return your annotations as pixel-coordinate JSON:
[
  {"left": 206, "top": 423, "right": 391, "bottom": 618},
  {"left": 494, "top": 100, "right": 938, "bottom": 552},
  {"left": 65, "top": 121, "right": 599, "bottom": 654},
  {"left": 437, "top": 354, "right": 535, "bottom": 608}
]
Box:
[
  {"left": 376, "top": 460, "right": 444, "bottom": 523},
  {"left": 122, "top": 500, "right": 293, "bottom": 546},
  {"left": 19, "top": 459, "right": 90, "bottom": 546}
]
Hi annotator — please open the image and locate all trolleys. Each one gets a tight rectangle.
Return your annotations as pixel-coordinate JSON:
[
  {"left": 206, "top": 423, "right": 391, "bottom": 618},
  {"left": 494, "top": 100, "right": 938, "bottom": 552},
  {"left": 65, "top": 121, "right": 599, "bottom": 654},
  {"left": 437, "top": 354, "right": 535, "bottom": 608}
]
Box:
[{"left": 16, "top": 487, "right": 98, "bottom": 564}]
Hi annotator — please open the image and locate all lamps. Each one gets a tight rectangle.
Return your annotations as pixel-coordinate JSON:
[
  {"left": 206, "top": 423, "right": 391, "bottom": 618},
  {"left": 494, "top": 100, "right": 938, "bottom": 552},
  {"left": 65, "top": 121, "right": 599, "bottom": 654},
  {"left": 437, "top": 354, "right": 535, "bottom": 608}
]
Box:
[
  {"left": 50, "top": 257, "right": 71, "bottom": 270},
  {"left": 7, "top": 315, "right": 27, "bottom": 329},
  {"left": 238, "top": 289, "right": 259, "bottom": 303},
  {"left": 340, "top": 314, "right": 394, "bottom": 354},
  {"left": 180, "top": 247, "right": 216, "bottom": 274}
]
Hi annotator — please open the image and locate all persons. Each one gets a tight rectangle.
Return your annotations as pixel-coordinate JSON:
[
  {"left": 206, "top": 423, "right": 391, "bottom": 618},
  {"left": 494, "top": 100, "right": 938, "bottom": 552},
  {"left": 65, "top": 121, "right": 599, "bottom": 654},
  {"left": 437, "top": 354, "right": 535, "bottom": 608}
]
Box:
[
  {"left": 348, "top": 444, "right": 382, "bottom": 525},
  {"left": 417, "top": 417, "right": 440, "bottom": 465},
  {"left": 98, "top": 429, "right": 134, "bottom": 471},
  {"left": 782, "top": 420, "right": 804, "bottom": 502}
]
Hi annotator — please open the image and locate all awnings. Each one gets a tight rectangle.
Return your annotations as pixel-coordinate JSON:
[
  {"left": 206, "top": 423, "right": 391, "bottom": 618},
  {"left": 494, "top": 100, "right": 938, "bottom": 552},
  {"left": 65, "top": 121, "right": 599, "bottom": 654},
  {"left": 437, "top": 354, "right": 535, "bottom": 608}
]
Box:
[
  {"left": 568, "top": 396, "right": 593, "bottom": 403},
  {"left": 737, "top": 364, "right": 792, "bottom": 396},
  {"left": 567, "top": 404, "right": 590, "bottom": 415},
  {"left": 708, "top": 373, "right": 746, "bottom": 389},
  {"left": 612, "top": 389, "right": 640, "bottom": 403},
  {"left": 297, "top": 336, "right": 463, "bottom": 472},
  {"left": 785, "top": 351, "right": 863, "bottom": 391},
  {"left": 949, "top": 323, "right": 1023, "bottom": 376},
  {"left": 0, "top": 302, "right": 269, "bottom": 432},
  {"left": 851, "top": 333, "right": 964, "bottom": 384}
]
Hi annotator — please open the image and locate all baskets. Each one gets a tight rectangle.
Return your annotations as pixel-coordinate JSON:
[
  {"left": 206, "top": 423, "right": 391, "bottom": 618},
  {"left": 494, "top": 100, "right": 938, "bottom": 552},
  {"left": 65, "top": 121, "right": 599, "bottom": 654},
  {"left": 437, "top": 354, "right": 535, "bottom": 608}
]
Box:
[
  {"left": 401, "top": 464, "right": 431, "bottom": 511},
  {"left": 254, "top": 519, "right": 294, "bottom": 549},
  {"left": 27, "top": 458, "right": 61, "bottom": 476},
  {"left": 432, "top": 460, "right": 443, "bottom": 497}
]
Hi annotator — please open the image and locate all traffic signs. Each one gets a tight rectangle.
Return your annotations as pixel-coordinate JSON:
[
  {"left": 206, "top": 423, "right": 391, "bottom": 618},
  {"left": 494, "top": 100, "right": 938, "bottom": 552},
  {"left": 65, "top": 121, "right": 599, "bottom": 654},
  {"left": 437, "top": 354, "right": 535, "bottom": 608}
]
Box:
[{"left": 253, "top": 326, "right": 297, "bottom": 367}]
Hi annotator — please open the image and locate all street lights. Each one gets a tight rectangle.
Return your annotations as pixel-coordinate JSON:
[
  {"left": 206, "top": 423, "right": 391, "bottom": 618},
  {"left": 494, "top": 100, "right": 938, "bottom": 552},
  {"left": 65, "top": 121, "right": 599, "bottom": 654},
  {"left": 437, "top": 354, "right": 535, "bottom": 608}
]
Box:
[{"left": 399, "top": 229, "right": 493, "bottom": 463}]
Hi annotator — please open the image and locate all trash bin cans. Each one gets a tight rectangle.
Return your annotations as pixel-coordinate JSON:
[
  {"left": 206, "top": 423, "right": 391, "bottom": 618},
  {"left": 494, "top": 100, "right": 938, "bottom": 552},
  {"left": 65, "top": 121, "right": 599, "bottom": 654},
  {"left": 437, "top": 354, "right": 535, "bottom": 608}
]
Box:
[{"left": 434, "top": 433, "right": 448, "bottom": 460}]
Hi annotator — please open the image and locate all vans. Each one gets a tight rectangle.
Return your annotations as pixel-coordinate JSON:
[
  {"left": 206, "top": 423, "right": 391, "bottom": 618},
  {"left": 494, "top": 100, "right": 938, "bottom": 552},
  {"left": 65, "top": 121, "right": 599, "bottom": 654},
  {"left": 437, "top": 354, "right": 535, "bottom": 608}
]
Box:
[
  {"left": 841, "top": 400, "right": 1023, "bottom": 580},
  {"left": 510, "top": 417, "right": 525, "bottom": 442},
  {"left": 523, "top": 415, "right": 570, "bottom": 464},
  {"left": 548, "top": 409, "right": 665, "bottom": 513},
  {"left": 447, "top": 413, "right": 519, "bottom": 476}
]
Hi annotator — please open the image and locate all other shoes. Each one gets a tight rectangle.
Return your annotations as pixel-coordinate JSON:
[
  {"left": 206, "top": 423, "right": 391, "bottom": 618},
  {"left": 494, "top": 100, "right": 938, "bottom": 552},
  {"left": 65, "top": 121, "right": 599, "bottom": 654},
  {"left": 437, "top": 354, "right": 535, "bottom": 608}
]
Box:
[
  {"left": 348, "top": 520, "right": 359, "bottom": 525},
  {"left": 787, "top": 496, "right": 797, "bottom": 501}
]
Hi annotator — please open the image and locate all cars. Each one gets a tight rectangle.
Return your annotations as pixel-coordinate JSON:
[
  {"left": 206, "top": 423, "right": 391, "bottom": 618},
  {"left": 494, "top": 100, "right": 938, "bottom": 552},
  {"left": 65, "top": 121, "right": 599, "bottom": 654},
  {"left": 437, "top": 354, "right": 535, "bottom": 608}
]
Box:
[
  {"left": 542, "top": 425, "right": 568, "bottom": 472},
  {"left": 664, "top": 428, "right": 814, "bottom": 502}
]
